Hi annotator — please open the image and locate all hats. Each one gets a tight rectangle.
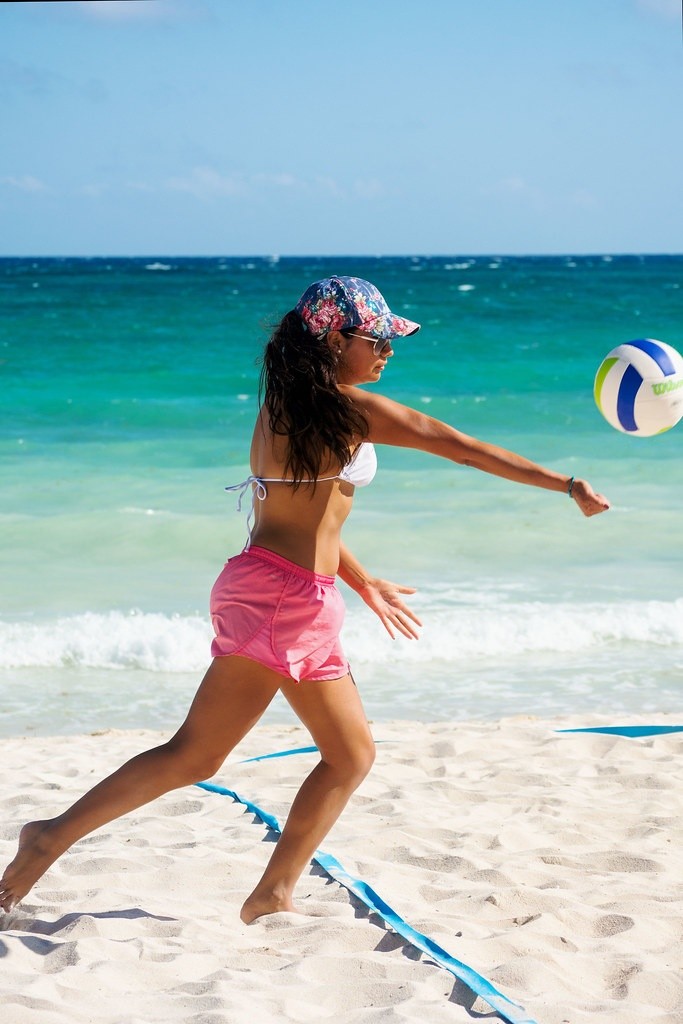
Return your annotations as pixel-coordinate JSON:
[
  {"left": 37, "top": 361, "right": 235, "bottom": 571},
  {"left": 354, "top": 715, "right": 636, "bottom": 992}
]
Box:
[{"left": 295, "top": 274, "right": 422, "bottom": 341}]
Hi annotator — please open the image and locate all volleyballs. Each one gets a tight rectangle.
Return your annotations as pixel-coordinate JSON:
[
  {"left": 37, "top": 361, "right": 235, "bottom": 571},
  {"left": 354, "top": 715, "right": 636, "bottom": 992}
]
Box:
[{"left": 593, "top": 337, "right": 683, "bottom": 439}]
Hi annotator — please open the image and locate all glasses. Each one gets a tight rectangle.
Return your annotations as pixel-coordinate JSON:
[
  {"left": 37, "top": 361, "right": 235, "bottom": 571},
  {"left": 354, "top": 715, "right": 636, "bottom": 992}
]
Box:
[{"left": 316, "top": 328, "right": 391, "bottom": 356}]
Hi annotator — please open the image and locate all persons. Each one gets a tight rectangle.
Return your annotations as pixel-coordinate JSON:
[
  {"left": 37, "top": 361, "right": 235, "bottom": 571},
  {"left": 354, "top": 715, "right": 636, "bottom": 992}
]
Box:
[{"left": 0, "top": 277, "right": 609, "bottom": 924}]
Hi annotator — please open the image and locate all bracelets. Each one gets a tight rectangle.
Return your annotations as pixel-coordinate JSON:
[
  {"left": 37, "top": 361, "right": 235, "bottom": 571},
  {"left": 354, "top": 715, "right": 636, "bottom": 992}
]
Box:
[{"left": 568, "top": 476, "right": 573, "bottom": 499}]
